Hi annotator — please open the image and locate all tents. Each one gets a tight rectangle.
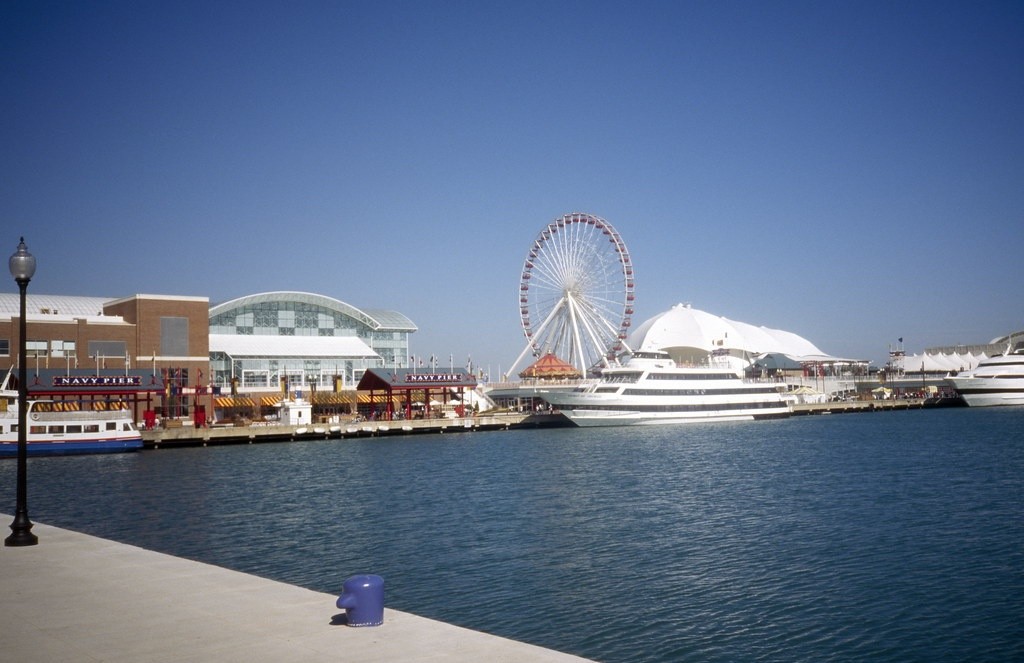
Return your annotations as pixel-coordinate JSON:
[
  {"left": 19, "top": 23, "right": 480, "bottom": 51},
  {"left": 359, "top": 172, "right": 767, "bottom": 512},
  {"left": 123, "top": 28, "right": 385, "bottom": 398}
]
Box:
[
  {"left": 871, "top": 387, "right": 892, "bottom": 399},
  {"left": 788, "top": 387, "right": 825, "bottom": 403}
]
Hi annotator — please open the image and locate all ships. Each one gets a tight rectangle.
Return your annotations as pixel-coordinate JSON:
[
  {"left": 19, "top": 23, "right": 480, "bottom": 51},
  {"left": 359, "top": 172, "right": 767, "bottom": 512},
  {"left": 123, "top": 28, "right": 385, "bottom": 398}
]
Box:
[{"left": 536, "top": 350, "right": 795, "bottom": 427}]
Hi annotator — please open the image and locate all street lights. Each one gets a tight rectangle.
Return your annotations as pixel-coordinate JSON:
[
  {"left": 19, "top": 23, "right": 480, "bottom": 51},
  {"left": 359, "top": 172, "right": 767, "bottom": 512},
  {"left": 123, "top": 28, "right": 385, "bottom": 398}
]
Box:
[{"left": 3, "top": 236, "right": 39, "bottom": 548}]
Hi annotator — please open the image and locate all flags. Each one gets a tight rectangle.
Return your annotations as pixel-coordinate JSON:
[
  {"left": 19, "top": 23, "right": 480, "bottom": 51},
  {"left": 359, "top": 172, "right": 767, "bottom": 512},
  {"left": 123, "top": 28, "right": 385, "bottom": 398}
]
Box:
[
  {"left": 391, "top": 355, "right": 433, "bottom": 366},
  {"left": 162, "top": 366, "right": 203, "bottom": 388}
]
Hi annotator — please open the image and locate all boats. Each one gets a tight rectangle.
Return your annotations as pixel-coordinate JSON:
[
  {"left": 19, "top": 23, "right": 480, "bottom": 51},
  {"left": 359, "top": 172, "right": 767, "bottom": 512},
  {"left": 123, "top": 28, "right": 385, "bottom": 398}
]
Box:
[
  {"left": 943, "top": 355, "right": 1024, "bottom": 407},
  {"left": 0, "top": 409, "right": 144, "bottom": 459}
]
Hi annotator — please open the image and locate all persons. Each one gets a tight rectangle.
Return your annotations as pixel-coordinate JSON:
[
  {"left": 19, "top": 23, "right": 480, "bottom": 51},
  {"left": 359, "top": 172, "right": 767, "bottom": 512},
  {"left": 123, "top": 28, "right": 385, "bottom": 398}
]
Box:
[
  {"left": 902, "top": 389, "right": 958, "bottom": 398},
  {"left": 510, "top": 403, "right": 553, "bottom": 414},
  {"left": 140, "top": 417, "right": 160, "bottom": 431},
  {"left": 372, "top": 405, "right": 462, "bottom": 421}
]
